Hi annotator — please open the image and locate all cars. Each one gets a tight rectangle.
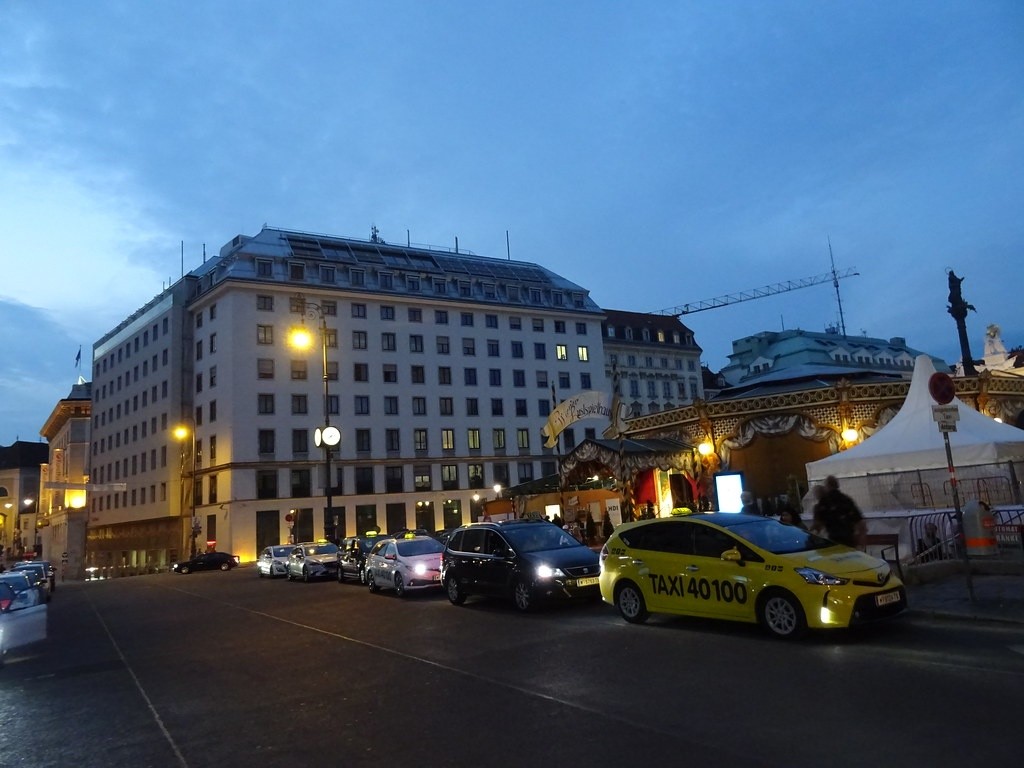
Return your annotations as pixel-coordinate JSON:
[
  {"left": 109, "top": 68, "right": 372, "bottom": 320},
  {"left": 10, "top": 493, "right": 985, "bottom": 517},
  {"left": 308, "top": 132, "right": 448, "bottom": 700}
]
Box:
[
  {"left": 335, "top": 528, "right": 452, "bottom": 586},
  {"left": 0, "top": 580, "right": 47, "bottom": 667},
  {"left": 172, "top": 552, "right": 241, "bottom": 576},
  {"left": 0, "top": 560, "right": 57, "bottom": 605},
  {"left": 364, "top": 532, "right": 447, "bottom": 598},
  {"left": 284, "top": 542, "right": 340, "bottom": 584},
  {"left": 255, "top": 544, "right": 296, "bottom": 579},
  {"left": 598, "top": 507, "right": 913, "bottom": 644}
]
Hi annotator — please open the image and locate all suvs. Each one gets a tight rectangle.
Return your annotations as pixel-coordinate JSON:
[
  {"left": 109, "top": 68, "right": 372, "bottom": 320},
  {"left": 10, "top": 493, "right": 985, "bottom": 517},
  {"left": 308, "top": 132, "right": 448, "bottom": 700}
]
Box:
[{"left": 440, "top": 517, "right": 600, "bottom": 614}]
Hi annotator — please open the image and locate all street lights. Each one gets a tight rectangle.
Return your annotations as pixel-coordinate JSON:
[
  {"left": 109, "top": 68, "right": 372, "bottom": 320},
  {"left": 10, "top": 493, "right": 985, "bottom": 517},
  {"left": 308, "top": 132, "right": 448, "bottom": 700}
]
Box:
[
  {"left": 171, "top": 417, "right": 198, "bottom": 561},
  {"left": 285, "top": 299, "right": 338, "bottom": 542},
  {"left": 23, "top": 491, "right": 39, "bottom": 553}
]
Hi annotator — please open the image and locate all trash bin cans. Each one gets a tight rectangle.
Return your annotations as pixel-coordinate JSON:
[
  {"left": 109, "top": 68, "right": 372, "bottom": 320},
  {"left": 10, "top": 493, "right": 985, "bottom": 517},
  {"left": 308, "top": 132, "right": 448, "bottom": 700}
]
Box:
[{"left": 961, "top": 497, "right": 1000, "bottom": 556}]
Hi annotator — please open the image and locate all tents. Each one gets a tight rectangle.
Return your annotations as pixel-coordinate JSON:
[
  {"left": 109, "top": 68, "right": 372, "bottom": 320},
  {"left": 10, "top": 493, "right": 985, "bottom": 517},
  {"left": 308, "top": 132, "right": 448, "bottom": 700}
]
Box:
[{"left": 805, "top": 354, "right": 1024, "bottom": 562}]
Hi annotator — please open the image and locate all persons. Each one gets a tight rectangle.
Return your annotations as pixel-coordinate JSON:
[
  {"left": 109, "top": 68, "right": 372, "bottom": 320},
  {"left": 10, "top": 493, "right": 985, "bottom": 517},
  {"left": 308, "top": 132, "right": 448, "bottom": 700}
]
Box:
[
  {"left": 780, "top": 505, "right": 809, "bottom": 530},
  {"left": 809, "top": 475, "right": 868, "bottom": 548},
  {"left": 552, "top": 514, "right": 562, "bottom": 528},
  {"left": 760, "top": 495, "right": 775, "bottom": 517},
  {"left": 916, "top": 522, "right": 944, "bottom": 564},
  {"left": 544, "top": 516, "right": 551, "bottom": 523}
]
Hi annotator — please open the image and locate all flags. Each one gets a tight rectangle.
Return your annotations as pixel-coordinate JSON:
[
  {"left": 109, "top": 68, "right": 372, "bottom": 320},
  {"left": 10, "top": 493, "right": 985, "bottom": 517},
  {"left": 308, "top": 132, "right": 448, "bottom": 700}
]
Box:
[{"left": 75, "top": 351, "right": 81, "bottom": 367}]
